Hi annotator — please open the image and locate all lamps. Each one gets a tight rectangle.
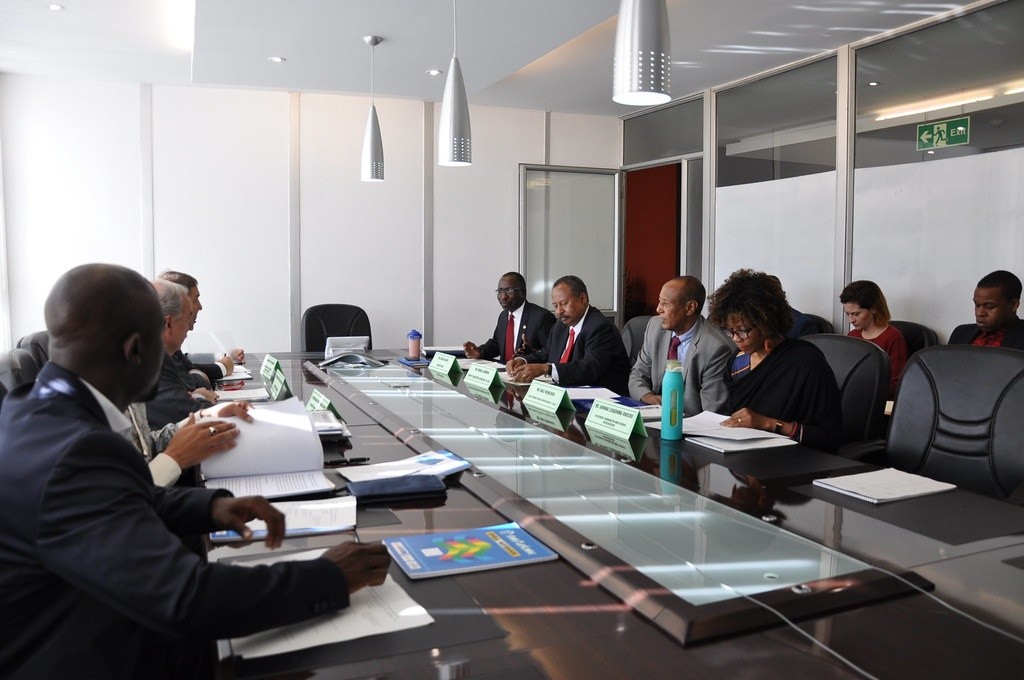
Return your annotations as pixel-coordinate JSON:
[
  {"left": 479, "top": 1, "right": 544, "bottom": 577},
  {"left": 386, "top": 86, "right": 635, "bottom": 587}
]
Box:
[
  {"left": 612, "top": 0, "right": 672, "bottom": 105},
  {"left": 360, "top": 36, "right": 384, "bottom": 182},
  {"left": 436, "top": 0, "right": 473, "bottom": 165}
]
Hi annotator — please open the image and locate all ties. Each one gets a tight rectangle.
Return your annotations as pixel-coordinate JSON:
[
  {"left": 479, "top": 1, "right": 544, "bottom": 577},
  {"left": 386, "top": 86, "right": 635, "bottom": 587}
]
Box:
[
  {"left": 505, "top": 314, "right": 515, "bottom": 362},
  {"left": 668, "top": 337, "right": 681, "bottom": 360},
  {"left": 560, "top": 329, "right": 575, "bottom": 363}
]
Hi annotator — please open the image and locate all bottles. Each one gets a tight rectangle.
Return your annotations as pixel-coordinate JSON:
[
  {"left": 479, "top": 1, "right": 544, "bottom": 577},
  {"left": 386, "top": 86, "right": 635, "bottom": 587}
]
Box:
[{"left": 407, "top": 329, "right": 422, "bottom": 361}]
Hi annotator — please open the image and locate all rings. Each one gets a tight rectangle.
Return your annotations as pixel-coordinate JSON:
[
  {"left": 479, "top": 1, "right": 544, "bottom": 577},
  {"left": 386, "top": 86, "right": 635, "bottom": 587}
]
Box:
[
  {"left": 209, "top": 426, "right": 217, "bottom": 436},
  {"left": 738, "top": 418, "right": 742, "bottom": 422}
]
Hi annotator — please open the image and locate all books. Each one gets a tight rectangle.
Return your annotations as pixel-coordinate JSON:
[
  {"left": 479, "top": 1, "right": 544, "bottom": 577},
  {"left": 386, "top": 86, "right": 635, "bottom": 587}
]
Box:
[
  {"left": 200, "top": 396, "right": 336, "bottom": 498},
  {"left": 382, "top": 521, "right": 558, "bottom": 579},
  {"left": 209, "top": 496, "right": 356, "bottom": 541}
]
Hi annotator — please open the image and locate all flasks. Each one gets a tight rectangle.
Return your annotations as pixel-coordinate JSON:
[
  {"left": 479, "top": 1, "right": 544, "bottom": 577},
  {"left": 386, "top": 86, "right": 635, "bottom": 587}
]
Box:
[{"left": 660, "top": 360, "right": 684, "bottom": 441}]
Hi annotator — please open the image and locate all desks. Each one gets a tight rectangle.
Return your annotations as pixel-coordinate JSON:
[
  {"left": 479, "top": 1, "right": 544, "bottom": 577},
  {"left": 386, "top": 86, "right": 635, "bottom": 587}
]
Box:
[{"left": 200, "top": 351, "right": 1024, "bottom": 679}]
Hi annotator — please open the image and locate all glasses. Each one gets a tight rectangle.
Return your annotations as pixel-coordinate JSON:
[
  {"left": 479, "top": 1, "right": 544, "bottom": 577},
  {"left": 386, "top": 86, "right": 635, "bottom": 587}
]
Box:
[
  {"left": 494, "top": 287, "right": 521, "bottom": 295},
  {"left": 719, "top": 326, "right": 755, "bottom": 339}
]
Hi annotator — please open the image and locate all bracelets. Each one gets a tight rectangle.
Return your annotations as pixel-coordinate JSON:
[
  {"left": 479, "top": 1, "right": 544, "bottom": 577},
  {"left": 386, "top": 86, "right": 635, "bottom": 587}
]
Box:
[
  {"left": 774, "top": 420, "right": 783, "bottom": 434},
  {"left": 790, "top": 421, "right": 800, "bottom": 441}
]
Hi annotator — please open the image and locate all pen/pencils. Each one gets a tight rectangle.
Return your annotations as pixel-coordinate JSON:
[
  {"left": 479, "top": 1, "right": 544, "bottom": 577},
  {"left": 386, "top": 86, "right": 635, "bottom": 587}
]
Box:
[
  {"left": 324, "top": 457, "right": 370, "bottom": 466},
  {"left": 465, "top": 345, "right": 476, "bottom": 347},
  {"left": 224, "top": 353, "right": 226, "bottom": 357},
  {"left": 512, "top": 355, "right": 514, "bottom": 377}
]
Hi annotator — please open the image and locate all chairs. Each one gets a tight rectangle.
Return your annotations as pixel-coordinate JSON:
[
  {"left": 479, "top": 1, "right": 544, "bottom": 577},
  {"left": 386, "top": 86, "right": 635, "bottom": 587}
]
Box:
[
  {"left": 884, "top": 344, "right": 1024, "bottom": 507},
  {"left": 888, "top": 320, "right": 938, "bottom": 363},
  {"left": 619, "top": 315, "right": 652, "bottom": 371},
  {"left": 301, "top": 304, "right": 372, "bottom": 352},
  {"left": 800, "top": 334, "right": 893, "bottom": 467}
]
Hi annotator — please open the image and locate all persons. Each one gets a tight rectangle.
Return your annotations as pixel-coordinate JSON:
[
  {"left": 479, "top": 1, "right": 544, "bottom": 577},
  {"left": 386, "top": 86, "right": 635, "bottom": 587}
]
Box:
[
  {"left": 643, "top": 425, "right": 720, "bottom": 503},
  {"left": 840, "top": 280, "right": 907, "bottom": 393},
  {"left": 496, "top": 388, "right": 531, "bottom": 442},
  {"left": 145, "top": 278, "right": 219, "bottom": 433},
  {"left": 628, "top": 275, "right": 733, "bottom": 416},
  {"left": 707, "top": 269, "right": 844, "bottom": 455},
  {"left": 550, "top": 411, "right": 591, "bottom": 466},
  {"left": 0, "top": 263, "right": 392, "bottom": 680},
  {"left": 160, "top": 271, "right": 245, "bottom": 380},
  {"left": 463, "top": 272, "right": 556, "bottom": 363},
  {"left": 948, "top": 270, "right": 1024, "bottom": 351},
  {"left": 506, "top": 275, "right": 632, "bottom": 398},
  {"left": 123, "top": 401, "right": 255, "bottom": 488},
  {"left": 709, "top": 445, "right": 837, "bottom": 554}
]
